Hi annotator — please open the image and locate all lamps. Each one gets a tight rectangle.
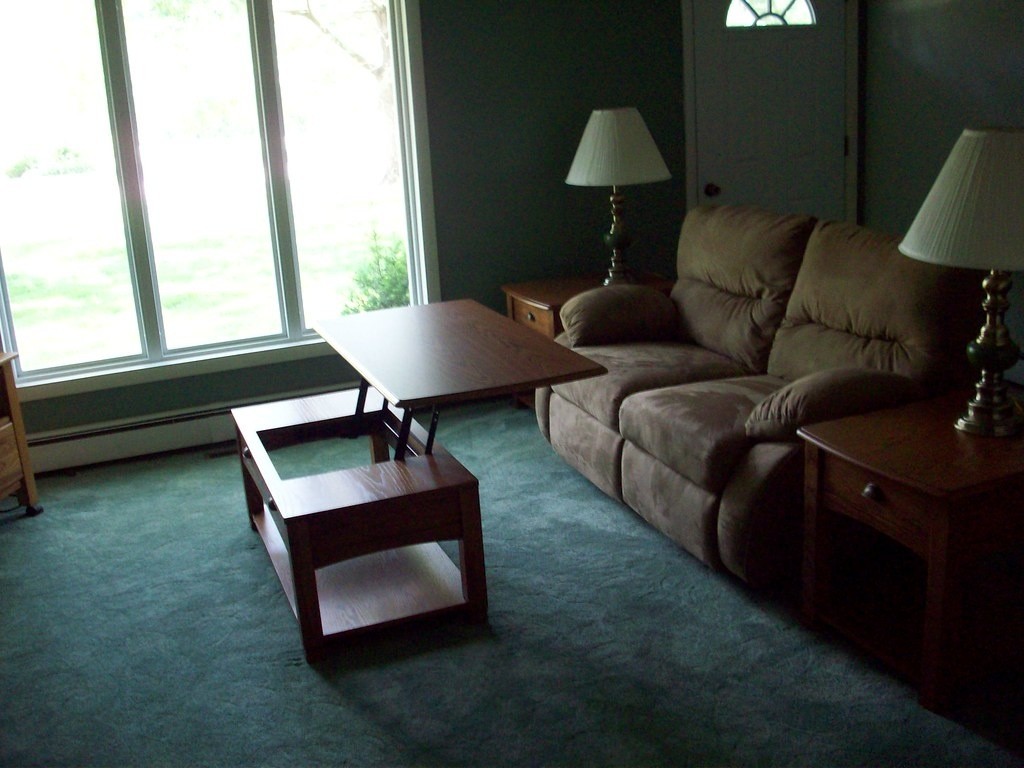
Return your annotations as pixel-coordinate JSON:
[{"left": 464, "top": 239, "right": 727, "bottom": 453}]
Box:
[
  {"left": 895, "top": 126, "right": 1022, "bottom": 439},
  {"left": 564, "top": 108, "right": 670, "bottom": 288}
]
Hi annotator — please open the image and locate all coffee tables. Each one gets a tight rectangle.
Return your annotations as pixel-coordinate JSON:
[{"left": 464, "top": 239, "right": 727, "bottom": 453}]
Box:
[{"left": 227, "top": 297, "right": 608, "bottom": 663}]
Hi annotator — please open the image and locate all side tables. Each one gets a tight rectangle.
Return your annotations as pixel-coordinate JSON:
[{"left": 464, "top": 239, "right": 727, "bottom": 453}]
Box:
[
  {"left": 797, "top": 398, "right": 1023, "bottom": 715},
  {"left": 501, "top": 262, "right": 671, "bottom": 409}
]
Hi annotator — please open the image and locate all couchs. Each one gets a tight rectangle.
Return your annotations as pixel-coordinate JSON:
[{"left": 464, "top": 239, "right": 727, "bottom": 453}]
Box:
[{"left": 532, "top": 204, "right": 949, "bottom": 592}]
